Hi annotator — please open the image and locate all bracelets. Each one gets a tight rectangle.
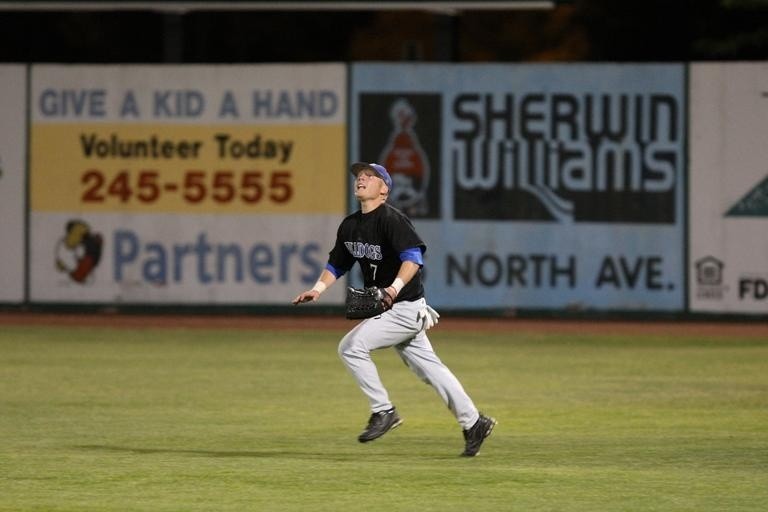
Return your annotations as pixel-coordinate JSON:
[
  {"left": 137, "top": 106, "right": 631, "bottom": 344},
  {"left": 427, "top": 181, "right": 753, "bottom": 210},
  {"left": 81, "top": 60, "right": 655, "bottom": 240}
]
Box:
[
  {"left": 313, "top": 281, "right": 327, "bottom": 294},
  {"left": 391, "top": 277, "right": 405, "bottom": 295}
]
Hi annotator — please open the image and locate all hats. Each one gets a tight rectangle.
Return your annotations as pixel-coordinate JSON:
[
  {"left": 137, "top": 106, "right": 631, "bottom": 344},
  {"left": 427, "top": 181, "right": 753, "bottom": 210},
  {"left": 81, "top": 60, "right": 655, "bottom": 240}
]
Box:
[{"left": 349, "top": 161, "right": 394, "bottom": 192}]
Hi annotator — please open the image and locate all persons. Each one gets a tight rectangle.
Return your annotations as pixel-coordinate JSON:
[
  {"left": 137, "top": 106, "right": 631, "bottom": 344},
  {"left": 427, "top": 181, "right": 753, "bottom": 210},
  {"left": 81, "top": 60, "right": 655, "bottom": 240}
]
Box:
[{"left": 289, "top": 160, "right": 496, "bottom": 457}]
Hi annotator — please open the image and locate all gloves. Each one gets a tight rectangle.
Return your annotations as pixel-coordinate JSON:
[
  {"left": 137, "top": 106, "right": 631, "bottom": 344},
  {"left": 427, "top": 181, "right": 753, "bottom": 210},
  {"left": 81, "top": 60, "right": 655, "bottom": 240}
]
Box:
[{"left": 416, "top": 305, "right": 443, "bottom": 331}]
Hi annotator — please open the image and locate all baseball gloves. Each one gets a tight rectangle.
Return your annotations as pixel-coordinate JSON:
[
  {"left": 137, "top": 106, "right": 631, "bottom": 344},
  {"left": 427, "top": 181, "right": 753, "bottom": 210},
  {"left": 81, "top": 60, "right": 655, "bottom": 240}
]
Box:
[{"left": 344, "top": 285, "right": 394, "bottom": 319}]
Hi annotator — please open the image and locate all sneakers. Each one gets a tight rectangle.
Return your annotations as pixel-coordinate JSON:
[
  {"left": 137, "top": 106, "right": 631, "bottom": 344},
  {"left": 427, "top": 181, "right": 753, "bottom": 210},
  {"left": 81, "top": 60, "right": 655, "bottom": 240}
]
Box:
[
  {"left": 458, "top": 411, "right": 499, "bottom": 459},
  {"left": 356, "top": 405, "right": 405, "bottom": 444}
]
